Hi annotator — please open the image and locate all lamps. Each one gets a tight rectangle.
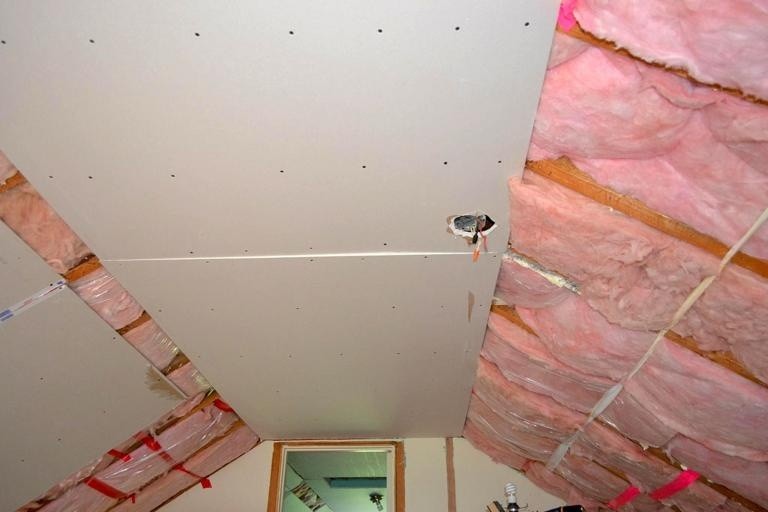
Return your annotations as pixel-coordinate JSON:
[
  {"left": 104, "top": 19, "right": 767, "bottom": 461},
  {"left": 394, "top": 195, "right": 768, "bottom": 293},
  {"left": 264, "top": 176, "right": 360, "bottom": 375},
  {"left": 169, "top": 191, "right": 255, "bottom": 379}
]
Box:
[
  {"left": 369, "top": 492, "right": 384, "bottom": 511},
  {"left": 503, "top": 481, "right": 520, "bottom": 512}
]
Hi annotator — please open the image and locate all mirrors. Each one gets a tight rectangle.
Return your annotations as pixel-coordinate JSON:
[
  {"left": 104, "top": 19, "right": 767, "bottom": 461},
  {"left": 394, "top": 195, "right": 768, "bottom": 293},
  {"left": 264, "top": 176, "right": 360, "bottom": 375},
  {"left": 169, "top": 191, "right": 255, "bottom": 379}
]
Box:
[{"left": 266, "top": 439, "right": 405, "bottom": 512}]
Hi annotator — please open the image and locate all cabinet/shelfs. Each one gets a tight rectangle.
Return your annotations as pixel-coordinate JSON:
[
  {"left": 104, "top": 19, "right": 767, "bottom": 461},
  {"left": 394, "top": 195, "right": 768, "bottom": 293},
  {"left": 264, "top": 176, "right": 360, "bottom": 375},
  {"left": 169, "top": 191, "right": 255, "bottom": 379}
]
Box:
[{"left": 282, "top": 480, "right": 334, "bottom": 512}]
